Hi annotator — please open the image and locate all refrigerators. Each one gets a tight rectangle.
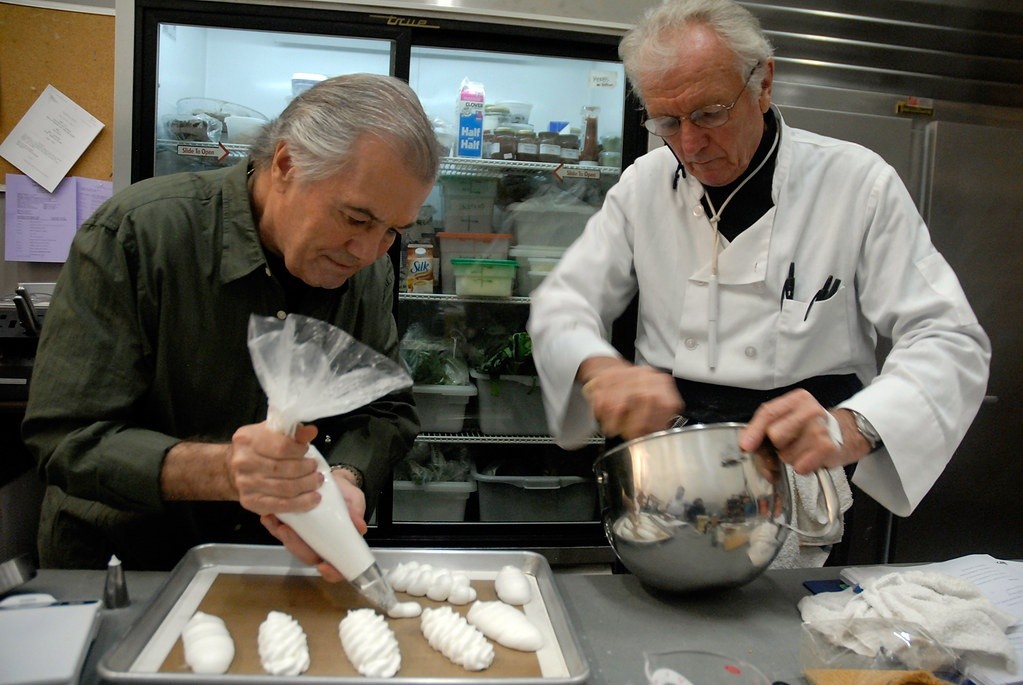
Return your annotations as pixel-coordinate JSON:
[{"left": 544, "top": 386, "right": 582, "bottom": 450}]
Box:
[{"left": 131, "top": 0, "right": 647, "bottom": 570}]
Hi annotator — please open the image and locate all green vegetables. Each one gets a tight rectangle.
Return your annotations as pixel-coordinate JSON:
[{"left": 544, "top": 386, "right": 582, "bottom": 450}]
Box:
[{"left": 405, "top": 332, "right": 531, "bottom": 396}]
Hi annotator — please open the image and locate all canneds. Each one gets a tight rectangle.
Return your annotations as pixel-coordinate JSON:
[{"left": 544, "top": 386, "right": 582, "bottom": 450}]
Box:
[{"left": 483, "top": 105, "right": 580, "bottom": 164}]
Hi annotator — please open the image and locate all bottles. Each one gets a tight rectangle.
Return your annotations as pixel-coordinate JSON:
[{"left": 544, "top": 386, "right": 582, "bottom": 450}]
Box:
[
  {"left": 538, "top": 131, "right": 561, "bottom": 164},
  {"left": 560, "top": 134, "right": 579, "bottom": 165},
  {"left": 579, "top": 114, "right": 600, "bottom": 160},
  {"left": 598, "top": 135, "right": 622, "bottom": 167},
  {"left": 483, "top": 104, "right": 537, "bottom": 160}
]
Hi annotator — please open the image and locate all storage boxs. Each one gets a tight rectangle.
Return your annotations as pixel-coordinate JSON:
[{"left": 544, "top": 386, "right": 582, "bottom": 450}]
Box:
[{"left": 392, "top": 175, "right": 598, "bottom": 520}]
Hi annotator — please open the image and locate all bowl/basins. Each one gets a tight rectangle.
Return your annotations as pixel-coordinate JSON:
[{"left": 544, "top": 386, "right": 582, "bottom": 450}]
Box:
[
  {"left": 224, "top": 116, "right": 270, "bottom": 145},
  {"left": 593, "top": 421, "right": 792, "bottom": 596}
]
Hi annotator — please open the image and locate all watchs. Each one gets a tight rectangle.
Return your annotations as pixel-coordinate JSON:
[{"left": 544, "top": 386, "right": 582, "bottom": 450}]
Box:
[{"left": 847, "top": 409, "right": 884, "bottom": 453}]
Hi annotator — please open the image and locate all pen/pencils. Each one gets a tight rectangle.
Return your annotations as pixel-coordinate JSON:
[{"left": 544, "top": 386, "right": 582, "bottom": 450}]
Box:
[
  {"left": 708, "top": 274, "right": 718, "bottom": 368},
  {"left": 780, "top": 262, "right": 795, "bottom": 311},
  {"left": 827, "top": 279, "right": 841, "bottom": 298},
  {"left": 804, "top": 275, "right": 833, "bottom": 320}
]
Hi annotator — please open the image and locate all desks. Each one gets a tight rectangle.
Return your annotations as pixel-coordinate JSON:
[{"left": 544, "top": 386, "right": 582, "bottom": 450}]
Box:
[{"left": 0, "top": 559, "right": 1023, "bottom": 685}]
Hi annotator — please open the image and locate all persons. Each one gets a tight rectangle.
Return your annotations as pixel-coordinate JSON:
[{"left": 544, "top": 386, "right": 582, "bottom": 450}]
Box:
[
  {"left": 527, "top": 0, "right": 994, "bottom": 571},
  {"left": 21, "top": 73, "right": 440, "bottom": 615}
]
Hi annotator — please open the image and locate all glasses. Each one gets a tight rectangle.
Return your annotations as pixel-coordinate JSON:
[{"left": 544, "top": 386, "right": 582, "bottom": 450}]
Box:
[{"left": 640, "top": 61, "right": 760, "bottom": 136}]
